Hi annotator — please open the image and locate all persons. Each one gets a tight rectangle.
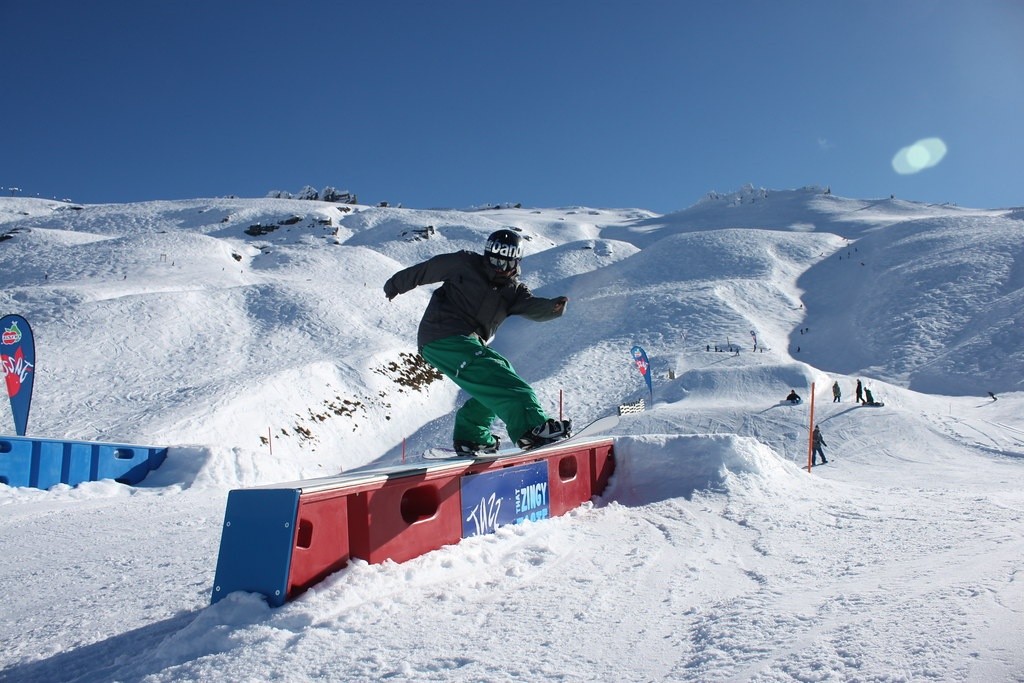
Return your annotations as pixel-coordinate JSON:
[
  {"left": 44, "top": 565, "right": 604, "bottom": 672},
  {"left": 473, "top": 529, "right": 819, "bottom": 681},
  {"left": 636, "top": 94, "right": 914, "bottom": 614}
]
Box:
[
  {"left": 707, "top": 345, "right": 710, "bottom": 351},
  {"left": 730, "top": 347, "right": 732, "bottom": 352},
  {"left": 812, "top": 425, "right": 828, "bottom": 465},
  {"left": 856, "top": 379, "right": 873, "bottom": 403},
  {"left": 988, "top": 391, "right": 997, "bottom": 401},
  {"left": 759, "top": 344, "right": 763, "bottom": 353},
  {"left": 715, "top": 346, "right": 717, "bottom": 352},
  {"left": 753, "top": 344, "right": 756, "bottom": 352},
  {"left": 833, "top": 381, "right": 841, "bottom": 402},
  {"left": 383, "top": 229, "right": 572, "bottom": 456},
  {"left": 787, "top": 390, "right": 801, "bottom": 405},
  {"left": 735, "top": 346, "right": 740, "bottom": 356},
  {"left": 718, "top": 349, "right": 722, "bottom": 352}
]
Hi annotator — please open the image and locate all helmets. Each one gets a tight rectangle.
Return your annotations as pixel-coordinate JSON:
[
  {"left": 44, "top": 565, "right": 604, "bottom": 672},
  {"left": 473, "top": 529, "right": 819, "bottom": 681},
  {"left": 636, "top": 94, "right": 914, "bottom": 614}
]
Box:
[{"left": 484, "top": 229, "right": 524, "bottom": 261}]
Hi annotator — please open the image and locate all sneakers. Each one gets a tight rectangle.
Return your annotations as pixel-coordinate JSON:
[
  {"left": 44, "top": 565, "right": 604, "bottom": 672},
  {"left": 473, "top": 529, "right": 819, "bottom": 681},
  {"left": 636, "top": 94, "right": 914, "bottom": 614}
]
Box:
[
  {"left": 517, "top": 417, "right": 573, "bottom": 452},
  {"left": 452, "top": 434, "right": 502, "bottom": 458}
]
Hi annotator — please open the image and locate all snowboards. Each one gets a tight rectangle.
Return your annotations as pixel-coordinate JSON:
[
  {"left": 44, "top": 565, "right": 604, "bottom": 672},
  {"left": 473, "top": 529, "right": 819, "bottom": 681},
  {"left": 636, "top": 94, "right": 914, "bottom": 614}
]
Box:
[{"left": 422, "top": 413, "right": 620, "bottom": 462}]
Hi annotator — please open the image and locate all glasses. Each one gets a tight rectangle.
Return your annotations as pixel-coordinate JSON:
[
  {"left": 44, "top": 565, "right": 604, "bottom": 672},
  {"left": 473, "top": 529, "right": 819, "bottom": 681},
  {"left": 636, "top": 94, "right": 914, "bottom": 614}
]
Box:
[{"left": 490, "top": 257, "right": 519, "bottom": 273}]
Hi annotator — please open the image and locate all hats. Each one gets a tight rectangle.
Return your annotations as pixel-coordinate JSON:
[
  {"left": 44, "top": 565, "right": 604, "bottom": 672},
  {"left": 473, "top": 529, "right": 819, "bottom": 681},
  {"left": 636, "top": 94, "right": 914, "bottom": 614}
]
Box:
[{"left": 815, "top": 425, "right": 819, "bottom": 429}]
